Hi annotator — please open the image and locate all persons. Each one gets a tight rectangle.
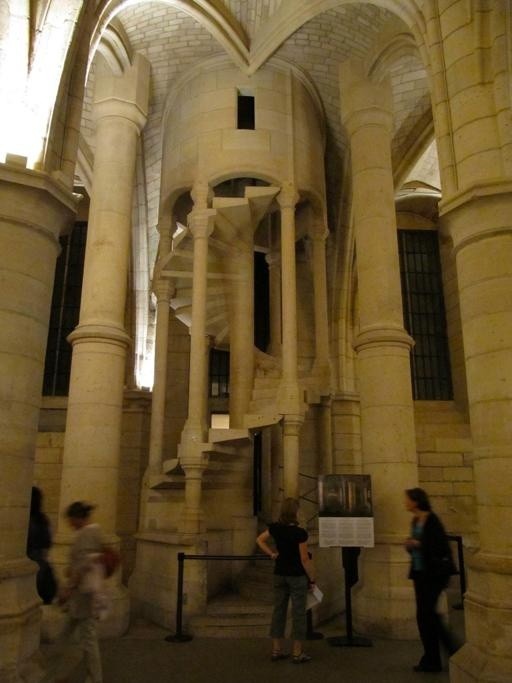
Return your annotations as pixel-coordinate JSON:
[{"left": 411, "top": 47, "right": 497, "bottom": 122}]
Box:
[
  {"left": 26, "top": 485, "right": 57, "bottom": 604},
  {"left": 400, "top": 488, "right": 459, "bottom": 671},
  {"left": 47, "top": 502, "right": 120, "bottom": 683},
  {"left": 257, "top": 498, "right": 316, "bottom": 664}
]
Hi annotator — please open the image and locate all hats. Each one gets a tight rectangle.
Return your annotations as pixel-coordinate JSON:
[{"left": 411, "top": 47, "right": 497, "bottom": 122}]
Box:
[{"left": 68, "top": 500, "right": 93, "bottom": 518}]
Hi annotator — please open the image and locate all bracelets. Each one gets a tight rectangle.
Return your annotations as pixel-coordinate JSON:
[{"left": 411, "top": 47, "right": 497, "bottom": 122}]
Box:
[{"left": 310, "top": 581, "right": 316, "bottom": 584}]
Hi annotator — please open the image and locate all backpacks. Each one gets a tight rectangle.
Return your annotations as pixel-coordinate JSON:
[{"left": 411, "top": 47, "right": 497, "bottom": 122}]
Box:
[{"left": 100, "top": 548, "right": 121, "bottom": 576}]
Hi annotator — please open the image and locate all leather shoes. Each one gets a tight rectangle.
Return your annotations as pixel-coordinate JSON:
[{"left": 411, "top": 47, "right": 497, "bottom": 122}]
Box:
[{"left": 412, "top": 663, "right": 443, "bottom": 673}]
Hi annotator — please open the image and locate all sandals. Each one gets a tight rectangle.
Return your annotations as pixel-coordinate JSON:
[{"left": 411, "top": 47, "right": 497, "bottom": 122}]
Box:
[{"left": 271, "top": 647, "right": 313, "bottom": 663}]
